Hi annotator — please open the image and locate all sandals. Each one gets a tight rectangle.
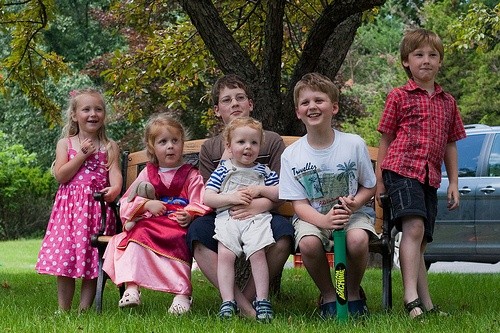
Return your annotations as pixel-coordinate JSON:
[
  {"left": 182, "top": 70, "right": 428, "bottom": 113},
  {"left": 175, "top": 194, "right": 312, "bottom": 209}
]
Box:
[
  {"left": 252, "top": 297, "right": 273, "bottom": 323},
  {"left": 425, "top": 304, "right": 452, "bottom": 318},
  {"left": 119, "top": 289, "right": 141, "bottom": 307},
  {"left": 219, "top": 299, "right": 237, "bottom": 320},
  {"left": 404, "top": 296, "right": 426, "bottom": 321},
  {"left": 167, "top": 295, "right": 192, "bottom": 315}
]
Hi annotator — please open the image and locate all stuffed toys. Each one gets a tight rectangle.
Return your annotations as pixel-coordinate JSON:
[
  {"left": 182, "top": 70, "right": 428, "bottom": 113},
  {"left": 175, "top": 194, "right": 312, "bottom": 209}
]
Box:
[{"left": 124, "top": 180, "right": 156, "bottom": 231}]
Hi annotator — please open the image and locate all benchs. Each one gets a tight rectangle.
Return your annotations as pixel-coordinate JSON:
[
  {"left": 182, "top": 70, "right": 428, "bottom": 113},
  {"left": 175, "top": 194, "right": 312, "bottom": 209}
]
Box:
[{"left": 91, "top": 136, "right": 391, "bottom": 313}]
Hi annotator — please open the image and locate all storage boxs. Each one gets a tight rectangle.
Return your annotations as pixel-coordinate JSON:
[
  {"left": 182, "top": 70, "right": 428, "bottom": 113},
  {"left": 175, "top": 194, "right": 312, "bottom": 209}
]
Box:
[{"left": 294, "top": 253, "right": 335, "bottom": 268}]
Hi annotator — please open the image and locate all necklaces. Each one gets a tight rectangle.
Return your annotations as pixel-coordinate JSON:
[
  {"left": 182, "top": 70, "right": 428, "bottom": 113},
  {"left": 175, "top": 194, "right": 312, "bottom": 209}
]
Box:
[{"left": 78, "top": 134, "right": 100, "bottom": 170}]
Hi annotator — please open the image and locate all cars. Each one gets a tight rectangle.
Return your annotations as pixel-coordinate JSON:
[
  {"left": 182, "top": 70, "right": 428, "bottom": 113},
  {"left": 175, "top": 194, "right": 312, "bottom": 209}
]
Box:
[{"left": 392, "top": 124, "right": 500, "bottom": 273}]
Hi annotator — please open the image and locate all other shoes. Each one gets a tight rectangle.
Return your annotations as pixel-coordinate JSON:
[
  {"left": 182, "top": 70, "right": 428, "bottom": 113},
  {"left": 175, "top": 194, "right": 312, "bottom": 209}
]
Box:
[
  {"left": 320, "top": 292, "right": 340, "bottom": 320},
  {"left": 347, "top": 287, "right": 367, "bottom": 318}
]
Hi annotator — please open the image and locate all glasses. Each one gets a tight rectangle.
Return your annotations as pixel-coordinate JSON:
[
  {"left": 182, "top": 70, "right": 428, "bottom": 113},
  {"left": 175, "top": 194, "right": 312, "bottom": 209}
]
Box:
[{"left": 217, "top": 94, "right": 248, "bottom": 104}]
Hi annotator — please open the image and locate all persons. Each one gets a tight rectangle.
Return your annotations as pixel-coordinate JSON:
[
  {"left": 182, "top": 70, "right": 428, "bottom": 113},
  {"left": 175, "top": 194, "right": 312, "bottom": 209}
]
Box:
[
  {"left": 35, "top": 88, "right": 123, "bottom": 313},
  {"left": 374, "top": 28, "right": 468, "bottom": 322},
  {"left": 101, "top": 112, "right": 213, "bottom": 314},
  {"left": 279, "top": 72, "right": 377, "bottom": 322},
  {"left": 185, "top": 74, "right": 294, "bottom": 320},
  {"left": 201, "top": 116, "right": 283, "bottom": 323}
]
